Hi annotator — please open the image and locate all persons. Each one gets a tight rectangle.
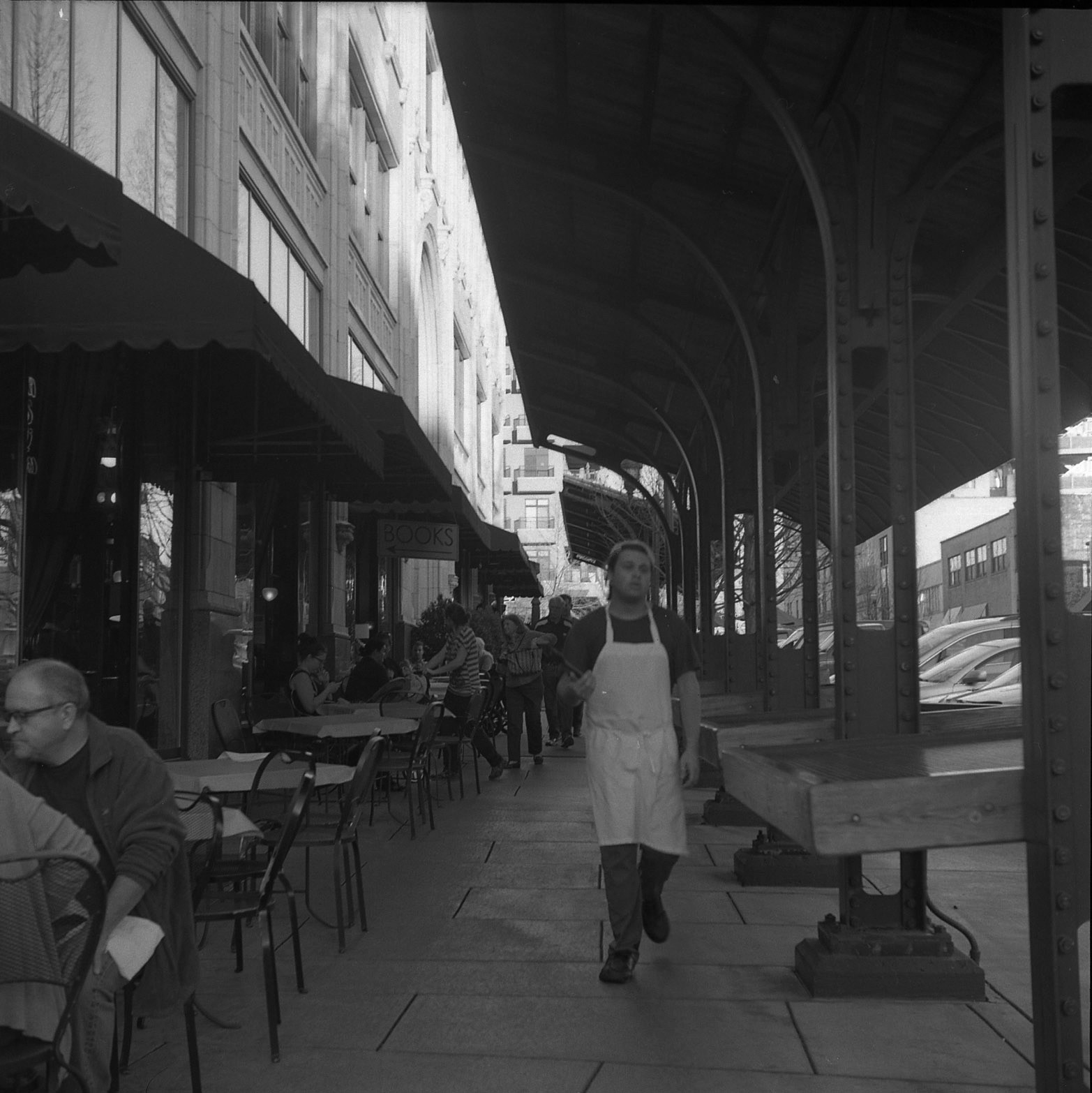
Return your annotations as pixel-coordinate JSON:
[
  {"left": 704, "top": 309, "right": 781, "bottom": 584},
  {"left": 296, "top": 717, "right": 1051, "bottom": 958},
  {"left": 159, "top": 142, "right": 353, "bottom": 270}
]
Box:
[
  {"left": 0, "top": 659, "right": 199, "bottom": 1093},
  {"left": 288, "top": 594, "right": 584, "bottom": 791},
  {"left": 556, "top": 540, "right": 701, "bottom": 983}
]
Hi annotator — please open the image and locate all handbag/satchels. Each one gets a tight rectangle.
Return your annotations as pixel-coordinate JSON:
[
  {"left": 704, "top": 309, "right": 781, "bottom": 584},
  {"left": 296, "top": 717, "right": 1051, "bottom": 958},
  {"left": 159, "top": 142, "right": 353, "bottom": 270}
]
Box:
[{"left": 506, "top": 631, "right": 543, "bottom": 677}]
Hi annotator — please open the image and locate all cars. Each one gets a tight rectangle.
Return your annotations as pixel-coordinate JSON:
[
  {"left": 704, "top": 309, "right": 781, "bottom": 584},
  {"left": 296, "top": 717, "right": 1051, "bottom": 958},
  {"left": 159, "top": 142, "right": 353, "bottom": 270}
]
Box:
[
  {"left": 920, "top": 638, "right": 1021, "bottom": 694},
  {"left": 920, "top": 661, "right": 1022, "bottom": 706},
  {"left": 776, "top": 623, "right": 888, "bottom": 685}
]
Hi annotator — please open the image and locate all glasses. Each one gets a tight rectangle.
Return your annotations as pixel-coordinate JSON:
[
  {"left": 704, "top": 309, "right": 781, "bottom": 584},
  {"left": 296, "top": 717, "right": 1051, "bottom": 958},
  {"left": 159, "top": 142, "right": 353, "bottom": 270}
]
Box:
[
  {"left": 312, "top": 656, "right": 324, "bottom": 664},
  {"left": 5, "top": 699, "right": 74, "bottom": 725}
]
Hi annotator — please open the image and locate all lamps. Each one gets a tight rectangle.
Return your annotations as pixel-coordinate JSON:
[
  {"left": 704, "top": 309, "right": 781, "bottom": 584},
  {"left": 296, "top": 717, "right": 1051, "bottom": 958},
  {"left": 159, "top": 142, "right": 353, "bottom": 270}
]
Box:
[{"left": 97, "top": 408, "right": 121, "bottom": 469}]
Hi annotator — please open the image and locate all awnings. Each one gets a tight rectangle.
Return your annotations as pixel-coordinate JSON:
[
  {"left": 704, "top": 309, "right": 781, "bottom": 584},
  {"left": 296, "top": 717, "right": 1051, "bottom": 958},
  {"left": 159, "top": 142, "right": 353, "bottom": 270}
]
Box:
[{"left": 0, "top": 101, "right": 543, "bottom": 597}]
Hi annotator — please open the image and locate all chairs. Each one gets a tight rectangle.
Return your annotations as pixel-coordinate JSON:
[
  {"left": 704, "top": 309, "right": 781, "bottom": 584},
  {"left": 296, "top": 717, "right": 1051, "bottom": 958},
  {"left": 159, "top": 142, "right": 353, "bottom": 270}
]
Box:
[{"left": 0, "top": 670, "right": 509, "bottom": 1093}]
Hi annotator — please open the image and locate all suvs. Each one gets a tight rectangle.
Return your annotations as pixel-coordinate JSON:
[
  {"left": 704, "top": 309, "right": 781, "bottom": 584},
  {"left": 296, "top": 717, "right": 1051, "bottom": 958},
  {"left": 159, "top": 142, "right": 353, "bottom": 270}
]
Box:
[{"left": 918, "top": 614, "right": 1021, "bottom": 672}]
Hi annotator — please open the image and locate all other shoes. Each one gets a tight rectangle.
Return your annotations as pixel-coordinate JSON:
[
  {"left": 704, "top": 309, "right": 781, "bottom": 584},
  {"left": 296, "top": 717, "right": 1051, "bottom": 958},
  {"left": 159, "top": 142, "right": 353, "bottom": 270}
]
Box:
[
  {"left": 383, "top": 782, "right": 405, "bottom": 792},
  {"left": 561, "top": 736, "right": 574, "bottom": 748},
  {"left": 534, "top": 754, "right": 544, "bottom": 763},
  {"left": 431, "top": 768, "right": 456, "bottom": 779},
  {"left": 642, "top": 892, "right": 669, "bottom": 943},
  {"left": 505, "top": 760, "right": 521, "bottom": 769},
  {"left": 489, "top": 759, "right": 507, "bottom": 780},
  {"left": 573, "top": 728, "right": 581, "bottom": 737},
  {"left": 598, "top": 949, "right": 636, "bottom": 982},
  {"left": 546, "top": 739, "right": 558, "bottom": 746}
]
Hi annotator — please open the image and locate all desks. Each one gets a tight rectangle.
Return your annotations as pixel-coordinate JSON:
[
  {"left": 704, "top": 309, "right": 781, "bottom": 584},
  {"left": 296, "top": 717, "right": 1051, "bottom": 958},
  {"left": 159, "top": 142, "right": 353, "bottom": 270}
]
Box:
[
  {"left": 254, "top": 715, "right": 419, "bottom": 821},
  {"left": 166, "top": 758, "right": 356, "bottom": 978},
  {"left": 346, "top": 702, "right": 454, "bottom": 787}
]
